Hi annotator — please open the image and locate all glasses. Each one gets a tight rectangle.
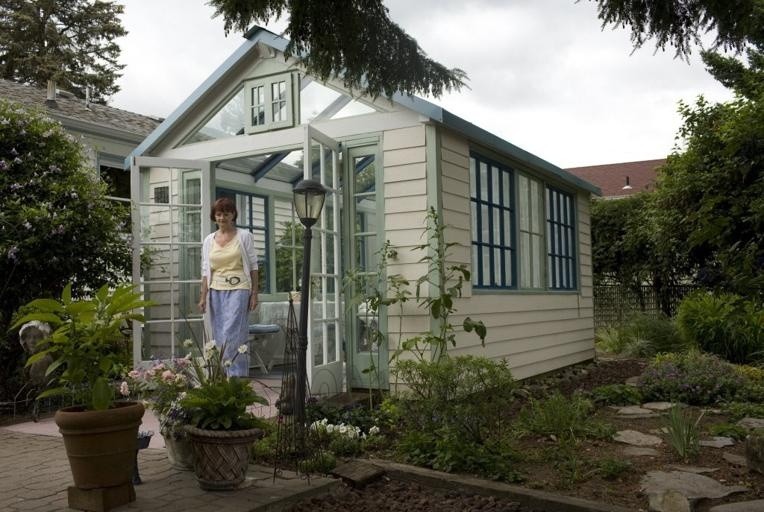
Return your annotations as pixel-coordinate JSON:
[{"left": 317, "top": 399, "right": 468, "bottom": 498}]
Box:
[{"left": 216, "top": 213, "right": 229, "bottom": 216}]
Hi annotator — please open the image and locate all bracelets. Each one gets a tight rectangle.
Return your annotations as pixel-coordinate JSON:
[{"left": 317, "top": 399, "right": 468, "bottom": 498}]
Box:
[{"left": 250, "top": 291, "right": 258, "bottom": 297}]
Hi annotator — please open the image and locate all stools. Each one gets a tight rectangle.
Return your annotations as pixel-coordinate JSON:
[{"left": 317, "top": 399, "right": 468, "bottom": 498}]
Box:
[{"left": 245, "top": 323, "right": 278, "bottom": 375}]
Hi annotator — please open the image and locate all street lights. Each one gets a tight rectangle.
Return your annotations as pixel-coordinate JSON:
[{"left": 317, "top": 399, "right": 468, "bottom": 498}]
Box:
[{"left": 292, "top": 180, "right": 327, "bottom": 450}]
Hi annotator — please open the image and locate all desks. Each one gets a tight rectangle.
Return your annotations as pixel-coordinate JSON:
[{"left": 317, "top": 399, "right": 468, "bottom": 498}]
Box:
[{"left": 256, "top": 300, "right": 353, "bottom": 370}]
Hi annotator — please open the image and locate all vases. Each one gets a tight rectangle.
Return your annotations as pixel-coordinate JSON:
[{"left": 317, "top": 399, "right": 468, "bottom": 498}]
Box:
[{"left": 161, "top": 428, "right": 191, "bottom": 469}]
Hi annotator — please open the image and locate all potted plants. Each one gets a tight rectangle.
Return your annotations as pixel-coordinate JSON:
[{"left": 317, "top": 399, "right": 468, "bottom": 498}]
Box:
[
  {"left": 180, "top": 377, "right": 270, "bottom": 492},
  {"left": 9, "top": 279, "right": 165, "bottom": 510},
  {"left": 290, "top": 285, "right": 303, "bottom": 302}
]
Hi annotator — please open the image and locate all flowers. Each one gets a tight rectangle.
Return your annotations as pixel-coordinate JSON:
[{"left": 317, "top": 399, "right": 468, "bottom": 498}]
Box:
[{"left": 116, "top": 335, "right": 249, "bottom": 430}]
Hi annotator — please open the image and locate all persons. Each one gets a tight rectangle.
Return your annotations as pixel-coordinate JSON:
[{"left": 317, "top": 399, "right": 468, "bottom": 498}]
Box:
[{"left": 195, "top": 195, "right": 262, "bottom": 380}]
